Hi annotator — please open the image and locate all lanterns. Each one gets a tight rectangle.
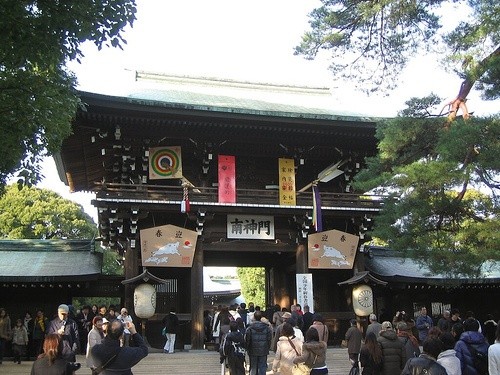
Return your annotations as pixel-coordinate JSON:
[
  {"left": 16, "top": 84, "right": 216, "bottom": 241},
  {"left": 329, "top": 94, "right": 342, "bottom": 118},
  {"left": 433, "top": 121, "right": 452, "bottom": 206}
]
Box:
[
  {"left": 352, "top": 284, "right": 373, "bottom": 317},
  {"left": 133, "top": 283, "right": 156, "bottom": 318}
]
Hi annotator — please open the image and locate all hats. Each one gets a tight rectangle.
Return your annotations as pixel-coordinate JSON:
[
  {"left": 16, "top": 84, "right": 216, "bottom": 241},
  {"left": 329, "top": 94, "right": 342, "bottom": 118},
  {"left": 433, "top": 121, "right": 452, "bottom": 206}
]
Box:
[
  {"left": 58, "top": 304, "right": 69, "bottom": 314},
  {"left": 281, "top": 312, "right": 291, "bottom": 318}
]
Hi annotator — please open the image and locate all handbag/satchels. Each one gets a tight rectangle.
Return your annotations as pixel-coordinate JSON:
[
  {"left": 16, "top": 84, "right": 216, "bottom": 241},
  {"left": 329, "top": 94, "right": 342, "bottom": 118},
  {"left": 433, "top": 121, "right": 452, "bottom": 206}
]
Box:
[
  {"left": 292, "top": 362, "right": 311, "bottom": 375},
  {"left": 161, "top": 327, "right": 167, "bottom": 336}
]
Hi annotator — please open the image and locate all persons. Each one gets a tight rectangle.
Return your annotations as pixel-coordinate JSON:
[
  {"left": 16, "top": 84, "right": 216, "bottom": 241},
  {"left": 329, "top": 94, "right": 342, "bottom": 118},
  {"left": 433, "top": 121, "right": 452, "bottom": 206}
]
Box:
[
  {"left": 223, "top": 321, "right": 248, "bottom": 375},
  {"left": 68, "top": 304, "right": 133, "bottom": 356},
  {"left": 0, "top": 308, "right": 12, "bottom": 363},
  {"left": 272, "top": 322, "right": 303, "bottom": 375},
  {"left": 30, "top": 334, "right": 71, "bottom": 375},
  {"left": 344, "top": 306, "right": 499, "bottom": 375},
  {"left": 86, "top": 316, "right": 105, "bottom": 375},
  {"left": 24, "top": 311, "right": 34, "bottom": 334},
  {"left": 245, "top": 311, "right": 272, "bottom": 375},
  {"left": 160, "top": 306, "right": 179, "bottom": 354},
  {"left": 46, "top": 303, "right": 80, "bottom": 363},
  {"left": 32, "top": 308, "right": 50, "bottom": 360},
  {"left": 11, "top": 318, "right": 28, "bottom": 365},
  {"left": 293, "top": 328, "right": 329, "bottom": 375},
  {"left": 87, "top": 320, "right": 151, "bottom": 375},
  {"left": 207, "top": 301, "right": 330, "bottom": 367},
  {"left": 102, "top": 318, "right": 109, "bottom": 337}
]
